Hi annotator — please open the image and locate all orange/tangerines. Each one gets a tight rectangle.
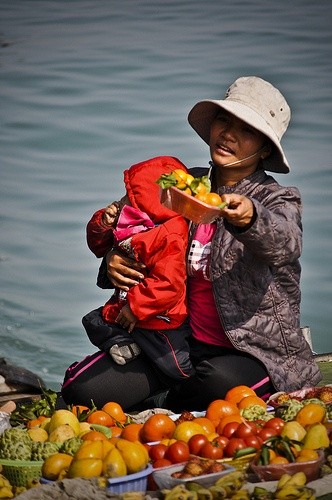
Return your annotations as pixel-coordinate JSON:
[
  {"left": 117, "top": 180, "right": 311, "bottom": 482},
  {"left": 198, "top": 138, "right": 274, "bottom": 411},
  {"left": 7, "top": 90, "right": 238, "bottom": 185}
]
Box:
[{"left": 71, "top": 385, "right": 266, "bottom": 444}]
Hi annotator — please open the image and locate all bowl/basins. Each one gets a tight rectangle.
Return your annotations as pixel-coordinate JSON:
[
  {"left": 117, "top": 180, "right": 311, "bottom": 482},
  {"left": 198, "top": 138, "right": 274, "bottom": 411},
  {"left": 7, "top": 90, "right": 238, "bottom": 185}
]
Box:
[
  {"left": 35, "top": 383, "right": 332, "bottom": 500},
  {"left": 158, "top": 184, "right": 229, "bottom": 223}
]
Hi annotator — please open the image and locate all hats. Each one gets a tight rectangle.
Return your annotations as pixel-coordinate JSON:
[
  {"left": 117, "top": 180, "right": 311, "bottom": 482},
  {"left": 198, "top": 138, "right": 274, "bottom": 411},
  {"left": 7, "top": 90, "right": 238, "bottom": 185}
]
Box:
[{"left": 188, "top": 76, "right": 292, "bottom": 174}]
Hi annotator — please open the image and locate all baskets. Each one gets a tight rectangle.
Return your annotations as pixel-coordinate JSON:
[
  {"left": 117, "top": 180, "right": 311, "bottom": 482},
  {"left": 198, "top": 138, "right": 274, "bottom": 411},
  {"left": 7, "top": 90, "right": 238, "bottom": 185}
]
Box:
[
  {"left": 159, "top": 185, "right": 223, "bottom": 226},
  {"left": 0, "top": 460, "right": 44, "bottom": 486},
  {"left": 39, "top": 462, "right": 154, "bottom": 494}
]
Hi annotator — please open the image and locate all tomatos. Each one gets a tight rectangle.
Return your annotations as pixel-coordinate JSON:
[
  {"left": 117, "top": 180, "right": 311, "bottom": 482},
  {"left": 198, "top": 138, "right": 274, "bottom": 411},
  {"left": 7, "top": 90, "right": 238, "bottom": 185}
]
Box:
[
  {"left": 28, "top": 402, "right": 330, "bottom": 481},
  {"left": 169, "top": 167, "right": 221, "bottom": 209}
]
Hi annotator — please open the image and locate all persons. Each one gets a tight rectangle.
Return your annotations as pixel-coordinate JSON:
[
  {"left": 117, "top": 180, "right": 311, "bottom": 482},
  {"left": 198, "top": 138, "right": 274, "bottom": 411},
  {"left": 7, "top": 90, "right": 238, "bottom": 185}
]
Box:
[
  {"left": 82, "top": 156, "right": 196, "bottom": 380},
  {"left": 61, "top": 75, "right": 323, "bottom": 412}
]
